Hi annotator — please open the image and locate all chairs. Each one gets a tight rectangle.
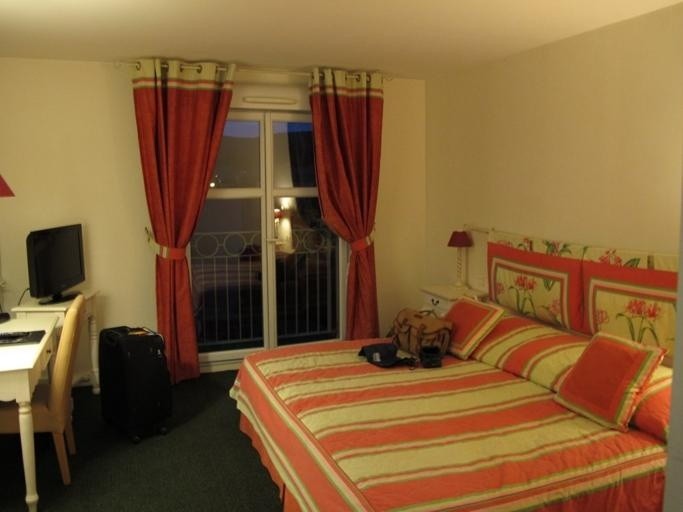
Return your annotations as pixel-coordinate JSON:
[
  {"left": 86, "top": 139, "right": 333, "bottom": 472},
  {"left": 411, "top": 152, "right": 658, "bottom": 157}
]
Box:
[{"left": 1, "top": 295, "right": 86, "bottom": 485}]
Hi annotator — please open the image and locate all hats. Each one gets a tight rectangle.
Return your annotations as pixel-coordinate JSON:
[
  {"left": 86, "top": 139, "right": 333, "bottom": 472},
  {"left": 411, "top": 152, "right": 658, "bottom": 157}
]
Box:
[{"left": 358, "top": 344, "right": 402, "bottom": 368}]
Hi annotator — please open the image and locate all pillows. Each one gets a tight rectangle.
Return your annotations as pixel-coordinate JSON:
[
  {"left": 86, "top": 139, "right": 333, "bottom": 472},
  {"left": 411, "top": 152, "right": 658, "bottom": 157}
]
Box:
[{"left": 442, "top": 295, "right": 671, "bottom": 447}]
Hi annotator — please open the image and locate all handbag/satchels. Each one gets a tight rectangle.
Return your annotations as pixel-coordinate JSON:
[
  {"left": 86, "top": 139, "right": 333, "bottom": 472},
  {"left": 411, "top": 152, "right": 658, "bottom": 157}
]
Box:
[{"left": 385, "top": 307, "right": 453, "bottom": 368}]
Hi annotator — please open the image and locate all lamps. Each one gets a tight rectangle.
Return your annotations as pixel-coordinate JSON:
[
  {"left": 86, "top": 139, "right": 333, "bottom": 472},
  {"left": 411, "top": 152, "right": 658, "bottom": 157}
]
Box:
[{"left": 447, "top": 231, "right": 474, "bottom": 289}]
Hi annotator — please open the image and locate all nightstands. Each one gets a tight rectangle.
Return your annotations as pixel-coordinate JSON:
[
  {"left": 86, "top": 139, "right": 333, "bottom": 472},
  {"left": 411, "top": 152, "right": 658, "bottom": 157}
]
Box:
[{"left": 418, "top": 284, "right": 488, "bottom": 319}]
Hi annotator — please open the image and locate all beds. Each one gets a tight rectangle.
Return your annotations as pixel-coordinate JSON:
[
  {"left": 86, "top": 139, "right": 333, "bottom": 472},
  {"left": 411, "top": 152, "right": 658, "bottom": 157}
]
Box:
[
  {"left": 242, "top": 228, "right": 678, "bottom": 512},
  {"left": 193, "top": 256, "right": 335, "bottom": 342}
]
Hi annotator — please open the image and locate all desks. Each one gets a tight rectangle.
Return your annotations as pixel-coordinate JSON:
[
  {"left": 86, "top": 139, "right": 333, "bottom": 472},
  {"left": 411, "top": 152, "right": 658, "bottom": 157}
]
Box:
[
  {"left": 0, "top": 314, "right": 78, "bottom": 511},
  {"left": 11, "top": 288, "right": 100, "bottom": 394}
]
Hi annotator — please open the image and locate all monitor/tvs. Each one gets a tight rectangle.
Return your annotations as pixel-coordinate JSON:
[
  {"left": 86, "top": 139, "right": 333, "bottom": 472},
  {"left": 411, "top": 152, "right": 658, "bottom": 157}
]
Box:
[{"left": 25, "top": 225, "right": 85, "bottom": 304}]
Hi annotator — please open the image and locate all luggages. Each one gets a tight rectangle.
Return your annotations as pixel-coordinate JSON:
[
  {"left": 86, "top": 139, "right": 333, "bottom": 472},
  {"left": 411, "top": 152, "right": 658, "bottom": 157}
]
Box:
[{"left": 98, "top": 326, "right": 174, "bottom": 445}]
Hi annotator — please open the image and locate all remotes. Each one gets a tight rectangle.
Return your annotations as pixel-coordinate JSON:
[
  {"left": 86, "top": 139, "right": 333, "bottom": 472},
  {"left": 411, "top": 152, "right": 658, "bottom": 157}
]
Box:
[{"left": 2, "top": 328, "right": 29, "bottom": 340}]
